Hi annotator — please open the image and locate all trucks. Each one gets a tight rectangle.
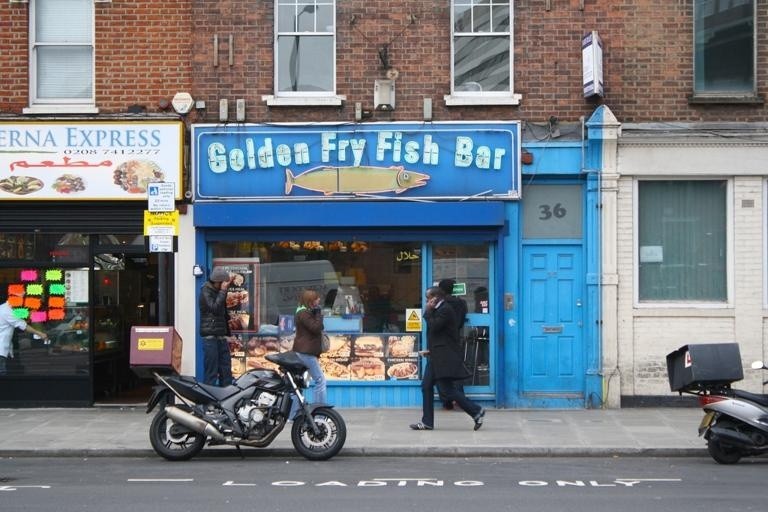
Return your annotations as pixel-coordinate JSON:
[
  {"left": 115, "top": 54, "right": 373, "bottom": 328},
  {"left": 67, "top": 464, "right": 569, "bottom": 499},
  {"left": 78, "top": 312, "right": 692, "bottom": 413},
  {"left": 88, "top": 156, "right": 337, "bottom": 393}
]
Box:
[{"left": 257, "top": 258, "right": 345, "bottom": 327}]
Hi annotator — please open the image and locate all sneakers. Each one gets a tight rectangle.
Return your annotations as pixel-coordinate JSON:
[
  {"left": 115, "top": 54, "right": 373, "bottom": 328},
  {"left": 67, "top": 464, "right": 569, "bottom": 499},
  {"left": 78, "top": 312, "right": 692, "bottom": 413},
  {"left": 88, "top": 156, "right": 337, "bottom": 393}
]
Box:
[
  {"left": 471, "top": 409, "right": 486, "bottom": 431},
  {"left": 408, "top": 422, "right": 434, "bottom": 431}
]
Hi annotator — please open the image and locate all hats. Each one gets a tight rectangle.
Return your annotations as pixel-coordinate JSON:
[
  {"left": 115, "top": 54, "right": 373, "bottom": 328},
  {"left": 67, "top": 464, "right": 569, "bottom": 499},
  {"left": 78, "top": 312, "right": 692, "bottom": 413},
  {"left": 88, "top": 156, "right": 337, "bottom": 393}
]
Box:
[{"left": 209, "top": 267, "right": 230, "bottom": 283}]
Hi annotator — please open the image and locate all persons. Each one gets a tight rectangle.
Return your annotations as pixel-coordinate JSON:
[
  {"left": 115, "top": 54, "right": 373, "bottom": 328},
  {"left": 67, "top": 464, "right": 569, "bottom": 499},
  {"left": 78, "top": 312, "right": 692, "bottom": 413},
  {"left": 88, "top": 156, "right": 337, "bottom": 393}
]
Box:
[
  {"left": 0, "top": 290, "right": 49, "bottom": 377},
  {"left": 408, "top": 286, "right": 489, "bottom": 432},
  {"left": 199, "top": 266, "right": 237, "bottom": 387},
  {"left": 285, "top": 287, "right": 327, "bottom": 424},
  {"left": 473, "top": 287, "right": 489, "bottom": 371},
  {"left": 437, "top": 279, "right": 470, "bottom": 410}
]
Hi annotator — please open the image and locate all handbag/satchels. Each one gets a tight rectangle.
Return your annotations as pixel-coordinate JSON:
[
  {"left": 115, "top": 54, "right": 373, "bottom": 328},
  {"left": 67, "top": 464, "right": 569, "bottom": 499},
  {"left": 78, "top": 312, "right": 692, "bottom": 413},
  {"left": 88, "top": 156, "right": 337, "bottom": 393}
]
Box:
[{"left": 319, "top": 328, "right": 330, "bottom": 354}]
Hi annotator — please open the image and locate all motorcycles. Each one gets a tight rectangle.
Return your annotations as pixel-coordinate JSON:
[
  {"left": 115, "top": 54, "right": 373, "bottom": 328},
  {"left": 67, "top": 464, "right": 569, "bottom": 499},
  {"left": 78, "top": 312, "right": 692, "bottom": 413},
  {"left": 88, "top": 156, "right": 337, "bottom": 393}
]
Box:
[
  {"left": 128, "top": 326, "right": 347, "bottom": 461},
  {"left": 666, "top": 343, "right": 768, "bottom": 468}
]
